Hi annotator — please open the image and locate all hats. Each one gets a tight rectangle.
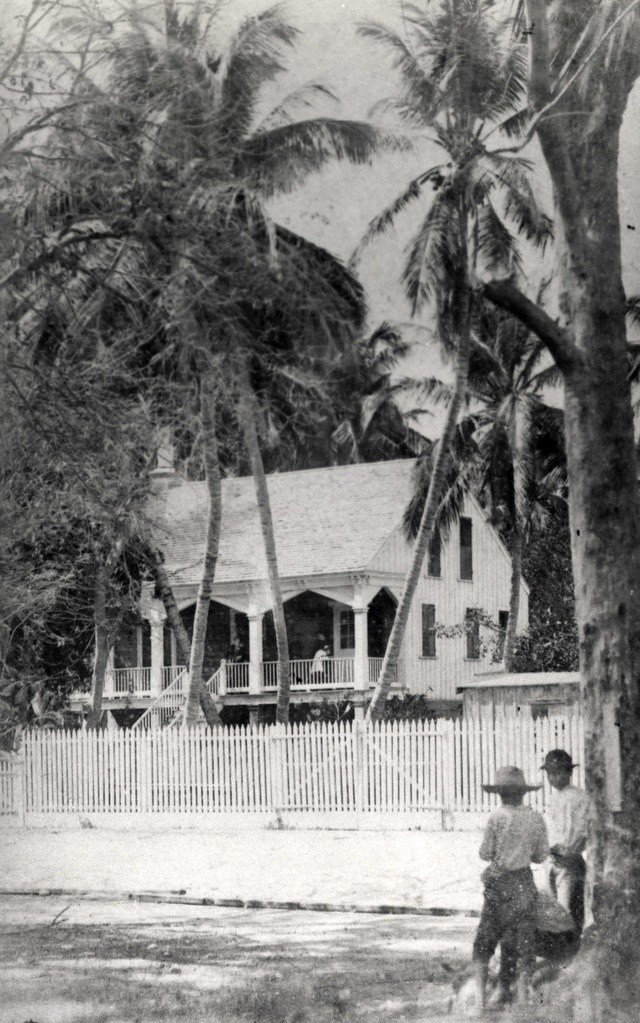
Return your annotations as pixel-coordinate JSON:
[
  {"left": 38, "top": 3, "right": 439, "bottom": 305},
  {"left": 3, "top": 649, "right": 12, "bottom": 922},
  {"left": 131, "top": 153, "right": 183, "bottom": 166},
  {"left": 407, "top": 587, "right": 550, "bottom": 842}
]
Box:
[
  {"left": 481, "top": 765, "right": 541, "bottom": 794},
  {"left": 539, "top": 749, "right": 580, "bottom": 770}
]
